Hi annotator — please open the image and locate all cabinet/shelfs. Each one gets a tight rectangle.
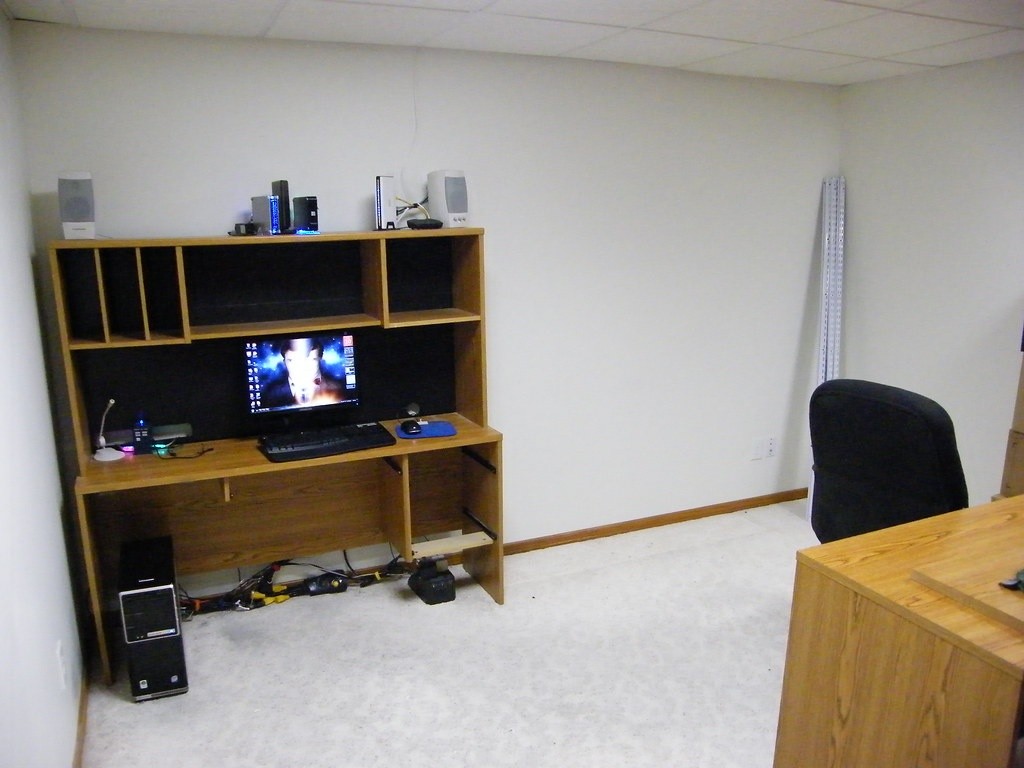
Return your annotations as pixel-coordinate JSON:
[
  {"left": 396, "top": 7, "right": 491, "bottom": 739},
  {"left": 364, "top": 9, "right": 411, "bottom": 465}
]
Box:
[{"left": 45, "top": 227, "right": 504, "bottom": 687}]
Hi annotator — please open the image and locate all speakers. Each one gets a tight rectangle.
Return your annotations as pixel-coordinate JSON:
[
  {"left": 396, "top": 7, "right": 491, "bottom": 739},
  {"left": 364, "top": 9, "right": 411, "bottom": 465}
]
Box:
[
  {"left": 58, "top": 173, "right": 95, "bottom": 240},
  {"left": 427, "top": 171, "right": 468, "bottom": 228}
]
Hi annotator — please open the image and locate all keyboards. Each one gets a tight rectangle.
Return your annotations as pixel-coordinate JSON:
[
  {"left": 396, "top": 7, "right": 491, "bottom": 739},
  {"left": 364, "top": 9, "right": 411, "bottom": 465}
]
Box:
[{"left": 258, "top": 422, "right": 396, "bottom": 463}]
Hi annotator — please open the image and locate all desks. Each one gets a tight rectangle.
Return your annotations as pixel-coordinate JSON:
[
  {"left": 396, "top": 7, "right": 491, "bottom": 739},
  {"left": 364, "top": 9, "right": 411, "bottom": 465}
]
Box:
[{"left": 772, "top": 493, "right": 1024, "bottom": 768}]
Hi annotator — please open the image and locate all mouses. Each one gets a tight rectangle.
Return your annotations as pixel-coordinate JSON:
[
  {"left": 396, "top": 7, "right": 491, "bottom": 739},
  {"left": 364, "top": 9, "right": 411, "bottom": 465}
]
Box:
[{"left": 402, "top": 420, "right": 421, "bottom": 434}]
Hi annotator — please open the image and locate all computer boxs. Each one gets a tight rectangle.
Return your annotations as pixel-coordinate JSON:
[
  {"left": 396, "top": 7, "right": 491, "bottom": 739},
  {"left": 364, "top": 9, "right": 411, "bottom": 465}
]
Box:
[{"left": 115, "top": 534, "right": 189, "bottom": 701}]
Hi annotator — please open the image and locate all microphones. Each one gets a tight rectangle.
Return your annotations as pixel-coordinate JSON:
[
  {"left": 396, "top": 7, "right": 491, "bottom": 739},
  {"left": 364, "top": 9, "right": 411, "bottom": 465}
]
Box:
[{"left": 95, "top": 399, "right": 125, "bottom": 461}]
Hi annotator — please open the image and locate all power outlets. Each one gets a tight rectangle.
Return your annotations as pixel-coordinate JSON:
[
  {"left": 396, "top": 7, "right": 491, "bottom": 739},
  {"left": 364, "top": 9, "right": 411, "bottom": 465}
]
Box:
[{"left": 751, "top": 434, "right": 778, "bottom": 460}]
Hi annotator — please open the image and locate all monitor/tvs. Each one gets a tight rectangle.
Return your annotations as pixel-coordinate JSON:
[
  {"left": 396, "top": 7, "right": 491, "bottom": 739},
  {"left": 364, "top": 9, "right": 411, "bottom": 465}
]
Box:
[{"left": 239, "top": 327, "right": 364, "bottom": 431}]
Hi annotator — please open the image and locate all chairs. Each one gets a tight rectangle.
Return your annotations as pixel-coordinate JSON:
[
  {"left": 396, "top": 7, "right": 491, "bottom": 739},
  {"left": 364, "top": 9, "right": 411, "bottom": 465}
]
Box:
[{"left": 809, "top": 378, "right": 969, "bottom": 543}]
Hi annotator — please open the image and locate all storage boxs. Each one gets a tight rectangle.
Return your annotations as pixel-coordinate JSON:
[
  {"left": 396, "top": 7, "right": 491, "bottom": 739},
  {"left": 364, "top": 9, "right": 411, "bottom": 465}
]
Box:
[{"left": 1000, "top": 429, "right": 1024, "bottom": 498}]
